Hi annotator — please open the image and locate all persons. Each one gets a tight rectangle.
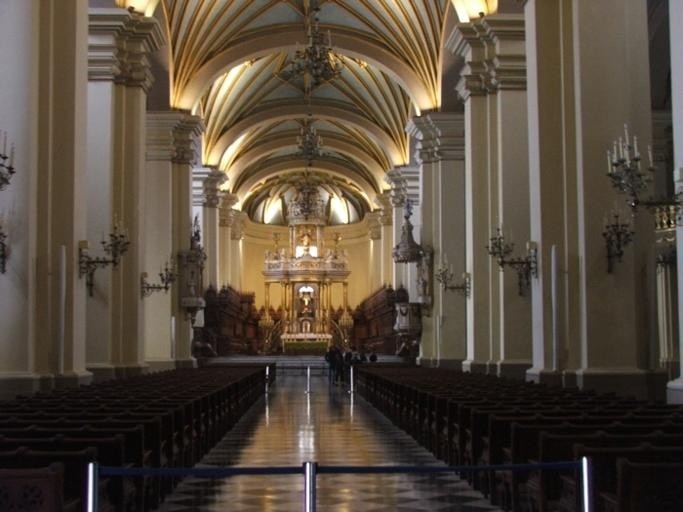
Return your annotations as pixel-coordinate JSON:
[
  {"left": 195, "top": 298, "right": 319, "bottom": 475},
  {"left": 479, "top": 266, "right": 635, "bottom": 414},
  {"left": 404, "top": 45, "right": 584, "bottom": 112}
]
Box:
[{"left": 325, "top": 345, "right": 378, "bottom": 387}]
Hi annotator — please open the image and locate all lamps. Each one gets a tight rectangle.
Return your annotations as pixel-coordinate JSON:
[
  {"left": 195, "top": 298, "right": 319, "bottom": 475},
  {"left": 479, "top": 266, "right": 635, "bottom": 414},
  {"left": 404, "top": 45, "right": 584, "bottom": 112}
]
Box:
[
  {"left": 604, "top": 119, "right": 683, "bottom": 227},
  {"left": 486, "top": 224, "right": 538, "bottom": 296},
  {"left": 0, "top": 129, "right": 16, "bottom": 274},
  {"left": 434, "top": 253, "right": 471, "bottom": 300},
  {"left": 140, "top": 250, "right": 177, "bottom": 300},
  {"left": 78, "top": 210, "right": 133, "bottom": 298},
  {"left": 600, "top": 208, "right": 635, "bottom": 278}
]
